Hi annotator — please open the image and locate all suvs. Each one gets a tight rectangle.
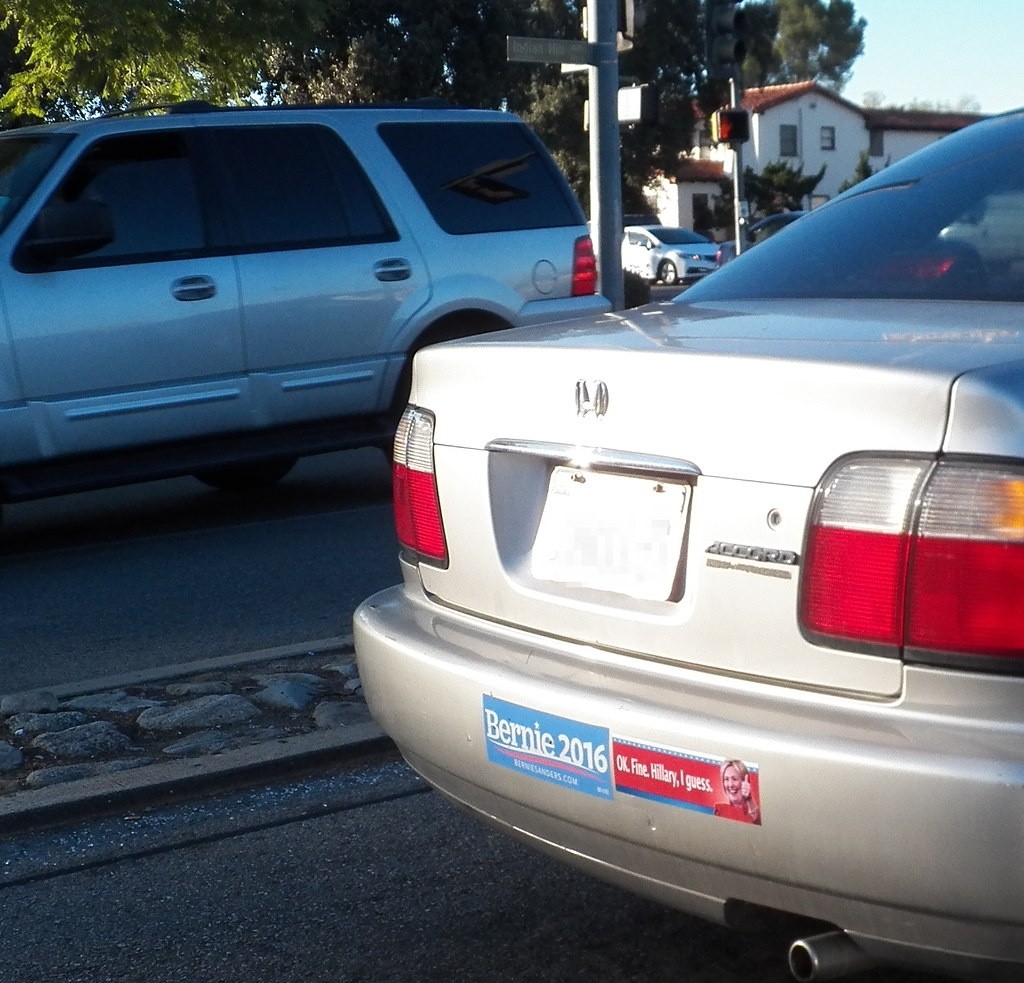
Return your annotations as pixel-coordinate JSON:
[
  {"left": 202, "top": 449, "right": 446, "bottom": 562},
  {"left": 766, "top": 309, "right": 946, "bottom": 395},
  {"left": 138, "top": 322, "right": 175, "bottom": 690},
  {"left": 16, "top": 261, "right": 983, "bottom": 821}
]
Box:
[{"left": 0, "top": 104, "right": 599, "bottom": 498}]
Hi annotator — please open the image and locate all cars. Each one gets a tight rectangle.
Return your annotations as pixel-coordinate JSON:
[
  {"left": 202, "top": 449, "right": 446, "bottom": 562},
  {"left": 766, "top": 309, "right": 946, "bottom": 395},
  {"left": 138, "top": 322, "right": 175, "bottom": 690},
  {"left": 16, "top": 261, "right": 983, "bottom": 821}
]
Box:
[
  {"left": 350, "top": 100, "right": 1024, "bottom": 983},
  {"left": 618, "top": 223, "right": 723, "bottom": 289},
  {"left": 716, "top": 211, "right": 811, "bottom": 270}
]
